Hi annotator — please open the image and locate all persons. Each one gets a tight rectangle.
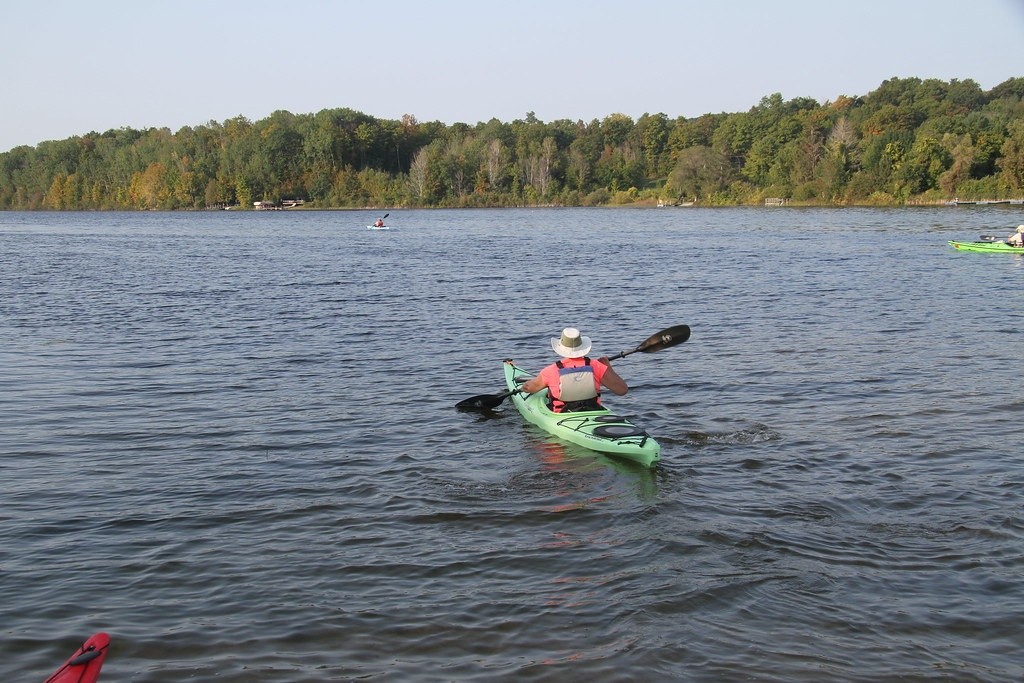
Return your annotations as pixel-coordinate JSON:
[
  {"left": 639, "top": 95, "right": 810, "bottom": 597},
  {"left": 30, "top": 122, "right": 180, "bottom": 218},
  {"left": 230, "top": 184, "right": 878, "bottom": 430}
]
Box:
[
  {"left": 1012, "top": 223, "right": 1023, "bottom": 247},
  {"left": 377, "top": 218, "right": 382, "bottom": 226},
  {"left": 521, "top": 327, "right": 627, "bottom": 412}
]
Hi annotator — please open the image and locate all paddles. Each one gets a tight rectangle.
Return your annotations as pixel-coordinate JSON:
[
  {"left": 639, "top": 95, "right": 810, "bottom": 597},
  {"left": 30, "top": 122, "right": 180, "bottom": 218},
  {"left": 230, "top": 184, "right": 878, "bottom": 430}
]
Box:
[
  {"left": 455, "top": 324, "right": 691, "bottom": 413},
  {"left": 372, "top": 213, "right": 389, "bottom": 226},
  {"left": 980, "top": 235, "right": 1010, "bottom": 241}
]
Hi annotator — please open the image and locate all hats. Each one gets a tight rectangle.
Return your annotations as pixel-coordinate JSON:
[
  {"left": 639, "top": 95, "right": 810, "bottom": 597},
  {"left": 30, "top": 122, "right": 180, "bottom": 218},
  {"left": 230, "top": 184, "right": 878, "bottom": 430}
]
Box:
[
  {"left": 377, "top": 218, "right": 382, "bottom": 221},
  {"left": 1016, "top": 225, "right": 1024, "bottom": 233},
  {"left": 550, "top": 327, "right": 592, "bottom": 358}
]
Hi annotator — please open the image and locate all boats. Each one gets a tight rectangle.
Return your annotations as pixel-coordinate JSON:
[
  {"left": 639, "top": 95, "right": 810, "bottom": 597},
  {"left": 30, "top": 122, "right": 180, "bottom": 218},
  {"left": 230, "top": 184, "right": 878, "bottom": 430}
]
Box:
[
  {"left": 501, "top": 356, "right": 663, "bottom": 467},
  {"left": 948, "top": 237, "right": 1024, "bottom": 259},
  {"left": 365, "top": 224, "right": 390, "bottom": 231}
]
